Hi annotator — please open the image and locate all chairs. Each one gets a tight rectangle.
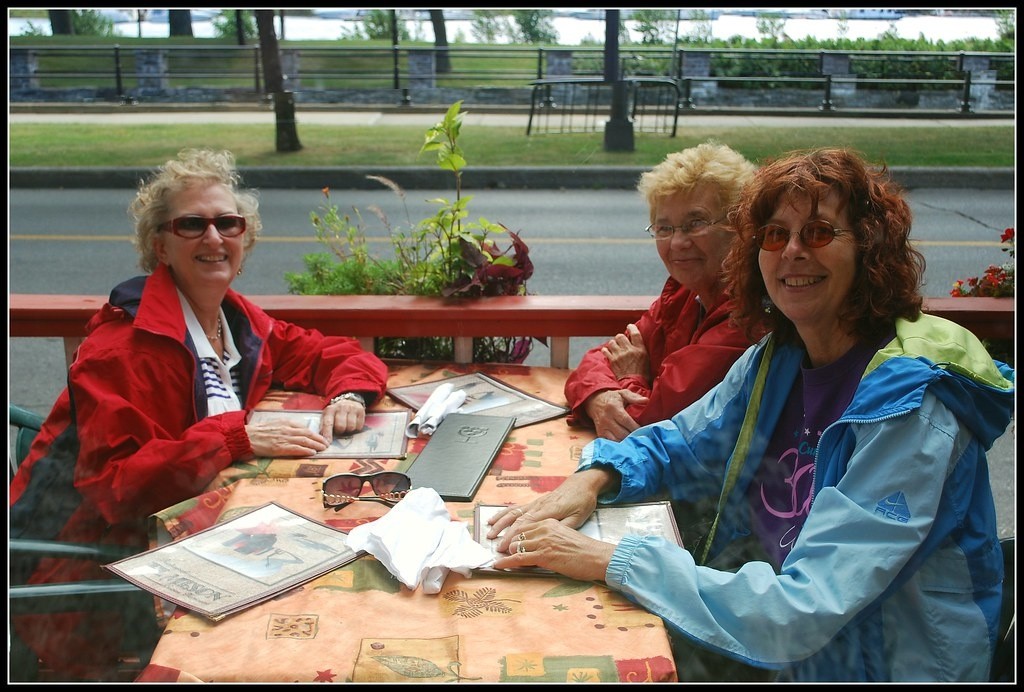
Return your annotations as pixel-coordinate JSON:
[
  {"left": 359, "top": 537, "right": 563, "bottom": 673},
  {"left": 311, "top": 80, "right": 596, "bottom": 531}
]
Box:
[{"left": 8, "top": 402, "right": 156, "bottom": 682}]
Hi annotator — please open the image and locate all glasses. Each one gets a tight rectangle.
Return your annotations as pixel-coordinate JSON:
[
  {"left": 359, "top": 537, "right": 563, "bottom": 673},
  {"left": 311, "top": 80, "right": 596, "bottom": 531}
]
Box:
[
  {"left": 157, "top": 214, "right": 246, "bottom": 239},
  {"left": 753, "top": 219, "right": 855, "bottom": 252},
  {"left": 644, "top": 215, "right": 726, "bottom": 237},
  {"left": 321, "top": 472, "right": 411, "bottom": 512}
]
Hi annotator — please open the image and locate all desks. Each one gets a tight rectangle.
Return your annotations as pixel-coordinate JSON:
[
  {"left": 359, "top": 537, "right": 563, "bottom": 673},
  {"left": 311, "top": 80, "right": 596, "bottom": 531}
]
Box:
[{"left": 137, "top": 360, "right": 679, "bottom": 682}]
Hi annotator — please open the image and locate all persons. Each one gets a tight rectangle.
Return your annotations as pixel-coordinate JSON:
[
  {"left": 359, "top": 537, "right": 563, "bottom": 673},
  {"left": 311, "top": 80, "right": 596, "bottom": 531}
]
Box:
[
  {"left": 561, "top": 138, "right": 772, "bottom": 443},
  {"left": 9, "top": 144, "right": 386, "bottom": 681},
  {"left": 488, "top": 146, "right": 1017, "bottom": 682}
]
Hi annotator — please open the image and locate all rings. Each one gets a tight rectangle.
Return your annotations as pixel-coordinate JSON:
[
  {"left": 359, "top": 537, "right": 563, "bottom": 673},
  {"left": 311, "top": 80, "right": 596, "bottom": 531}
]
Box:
[
  {"left": 525, "top": 512, "right": 537, "bottom": 520},
  {"left": 518, "top": 531, "right": 526, "bottom": 540},
  {"left": 516, "top": 542, "right": 526, "bottom": 553},
  {"left": 516, "top": 507, "right": 523, "bottom": 516}
]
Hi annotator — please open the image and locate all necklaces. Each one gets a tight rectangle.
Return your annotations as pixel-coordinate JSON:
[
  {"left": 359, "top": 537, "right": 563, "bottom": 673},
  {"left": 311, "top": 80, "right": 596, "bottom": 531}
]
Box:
[{"left": 206, "top": 318, "right": 222, "bottom": 341}]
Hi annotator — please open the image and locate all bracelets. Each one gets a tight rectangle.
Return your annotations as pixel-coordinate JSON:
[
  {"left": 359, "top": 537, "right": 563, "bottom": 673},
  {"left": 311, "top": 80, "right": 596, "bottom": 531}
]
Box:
[{"left": 330, "top": 392, "right": 365, "bottom": 404}]
